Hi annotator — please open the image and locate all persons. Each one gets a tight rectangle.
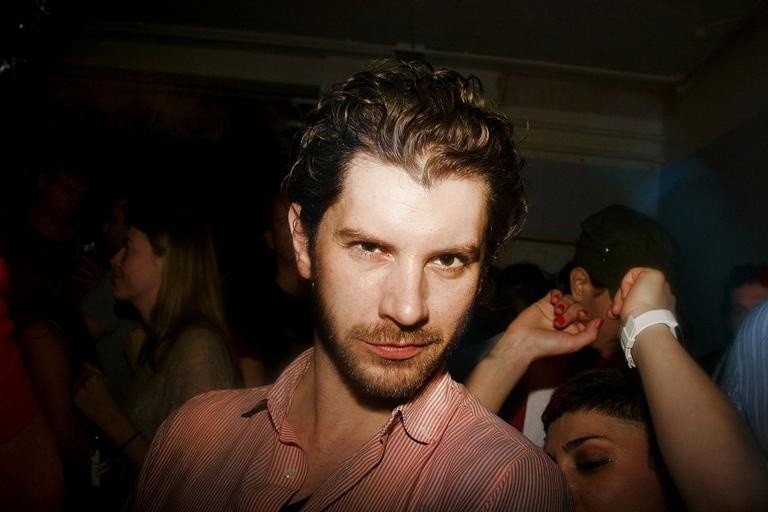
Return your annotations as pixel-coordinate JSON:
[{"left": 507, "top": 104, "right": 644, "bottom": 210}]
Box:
[
  {"left": 131, "top": 61, "right": 578, "bottom": 512},
  {"left": 1, "top": 129, "right": 768, "bottom": 510}
]
